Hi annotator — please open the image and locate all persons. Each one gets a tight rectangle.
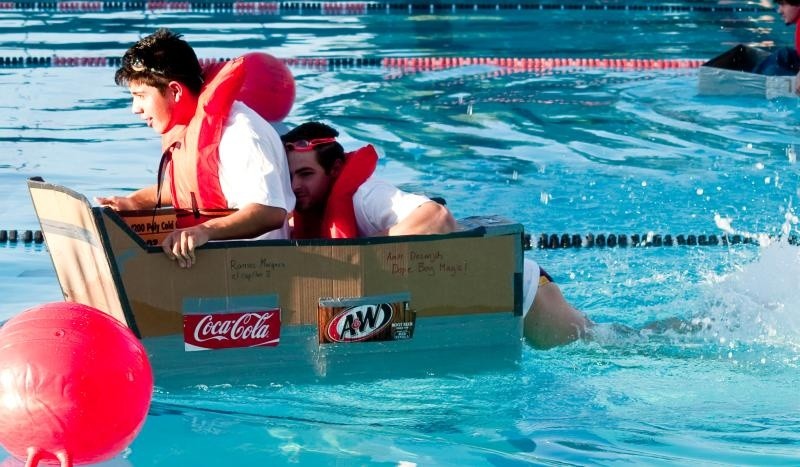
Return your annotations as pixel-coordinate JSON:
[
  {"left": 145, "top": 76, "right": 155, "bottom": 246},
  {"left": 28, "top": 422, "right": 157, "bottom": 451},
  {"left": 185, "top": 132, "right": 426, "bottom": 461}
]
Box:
[
  {"left": 773, "top": 0, "right": 800, "bottom": 95},
  {"left": 92, "top": 26, "right": 297, "bottom": 270},
  {"left": 278, "top": 120, "right": 586, "bottom": 348}
]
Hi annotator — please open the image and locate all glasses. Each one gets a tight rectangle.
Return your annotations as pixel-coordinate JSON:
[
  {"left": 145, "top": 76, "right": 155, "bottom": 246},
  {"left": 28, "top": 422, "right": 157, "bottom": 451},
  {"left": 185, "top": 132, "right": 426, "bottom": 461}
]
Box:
[
  {"left": 122, "top": 51, "right": 166, "bottom": 75},
  {"left": 282, "top": 140, "right": 313, "bottom": 152}
]
